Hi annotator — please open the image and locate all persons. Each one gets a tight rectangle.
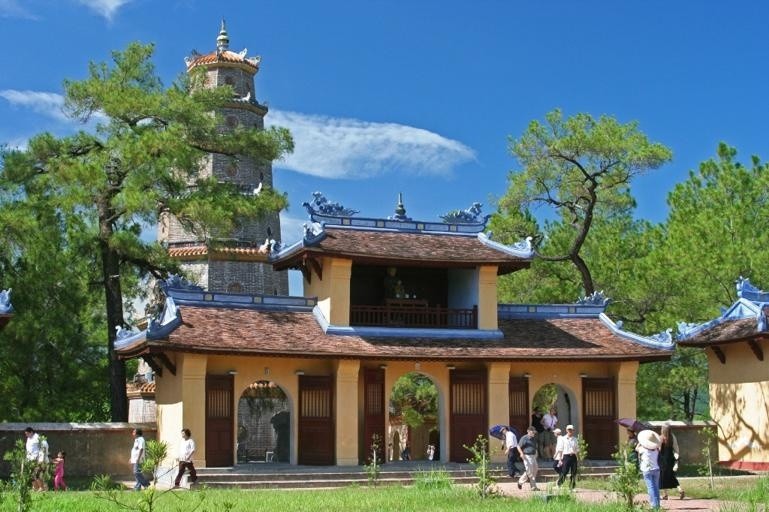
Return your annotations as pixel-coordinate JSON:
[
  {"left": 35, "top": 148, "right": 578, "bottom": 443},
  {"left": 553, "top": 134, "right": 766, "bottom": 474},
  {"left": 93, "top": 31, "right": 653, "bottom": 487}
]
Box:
[
  {"left": 515, "top": 424, "right": 543, "bottom": 492},
  {"left": 532, "top": 406, "right": 545, "bottom": 457},
  {"left": 556, "top": 424, "right": 581, "bottom": 493},
  {"left": 657, "top": 424, "right": 686, "bottom": 500},
  {"left": 50, "top": 451, "right": 69, "bottom": 492},
  {"left": 625, "top": 428, "right": 639, "bottom": 471},
  {"left": 33, "top": 433, "right": 52, "bottom": 491},
  {"left": 174, "top": 428, "right": 198, "bottom": 489},
  {"left": 127, "top": 428, "right": 151, "bottom": 491},
  {"left": 634, "top": 429, "right": 662, "bottom": 510},
  {"left": 499, "top": 427, "right": 520, "bottom": 479},
  {"left": 552, "top": 427, "right": 568, "bottom": 480},
  {"left": 23, "top": 425, "right": 42, "bottom": 492},
  {"left": 427, "top": 423, "right": 440, "bottom": 460},
  {"left": 382, "top": 264, "right": 408, "bottom": 299},
  {"left": 539, "top": 408, "right": 558, "bottom": 458}
]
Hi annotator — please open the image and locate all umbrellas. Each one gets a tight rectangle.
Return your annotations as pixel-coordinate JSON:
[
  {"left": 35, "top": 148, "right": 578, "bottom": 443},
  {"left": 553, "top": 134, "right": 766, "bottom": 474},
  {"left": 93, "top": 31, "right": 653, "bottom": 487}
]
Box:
[
  {"left": 489, "top": 424, "right": 520, "bottom": 449},
  {"left": 614, "top": 417, "right": 646, "bottom": 434}
]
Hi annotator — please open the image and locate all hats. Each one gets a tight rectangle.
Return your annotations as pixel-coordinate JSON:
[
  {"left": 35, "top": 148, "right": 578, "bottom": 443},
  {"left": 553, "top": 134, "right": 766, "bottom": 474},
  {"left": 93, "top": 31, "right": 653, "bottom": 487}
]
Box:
[
  {"left": 637, "top": 430, "right": 661, "bottom": 449},
  {"left": 500, "top": 426, "right": 508, "bottom": 432},
  {"left": 552, "top": 428, "right": 562, "bottom": 433},
  {"left": 566, "top": 425, "right": 574, "bottom": 430}
]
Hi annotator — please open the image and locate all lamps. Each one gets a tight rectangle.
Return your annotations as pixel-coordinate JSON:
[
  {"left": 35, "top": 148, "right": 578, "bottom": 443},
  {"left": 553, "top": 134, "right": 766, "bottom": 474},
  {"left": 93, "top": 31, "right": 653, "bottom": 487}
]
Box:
[
  {"left": 524, "top": 373, "right": 531, "bottom": 378},
  {"left": 380, "top": 364, "right": 387, "bottom": 368},
  {"left": 446, "top": 365, "right": 455, "bottom": 369},
  {"left": 229, "top": 370, "right": 238, "bottom": 375},
  {"left": 296, "top": 370, "right": 304, "bottom": 375},
  {"left": 580, "top": 372, "right": 588, "bottom": 377}
]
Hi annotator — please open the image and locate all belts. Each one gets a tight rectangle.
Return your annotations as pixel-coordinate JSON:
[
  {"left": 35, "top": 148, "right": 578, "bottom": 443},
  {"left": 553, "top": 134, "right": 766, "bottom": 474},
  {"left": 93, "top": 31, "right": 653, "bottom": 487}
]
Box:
[{"left": 564, "top": 454, "right": 575, "bottom": 457}]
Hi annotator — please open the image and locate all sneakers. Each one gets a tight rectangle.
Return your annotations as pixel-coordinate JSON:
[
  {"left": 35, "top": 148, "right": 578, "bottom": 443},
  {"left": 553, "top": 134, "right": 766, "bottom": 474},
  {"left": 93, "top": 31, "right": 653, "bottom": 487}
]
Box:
[
  {"left": 518, "top": 482, "right": 522, "bottom": 489},
  {"left": 663, "top": 497, "right": 668, "bottom": 500},
  {"left": 531, "top": 487, "right": 541, "bottom": 491},
  {"left": 680, "top": 491, "right": 685, "bottom": 499},
  {"left": 570, "top": 489, "right": 577, "bottom": 493},
  {"left": 556, "top": 480, "right": 561, "bottom": 489}
]
